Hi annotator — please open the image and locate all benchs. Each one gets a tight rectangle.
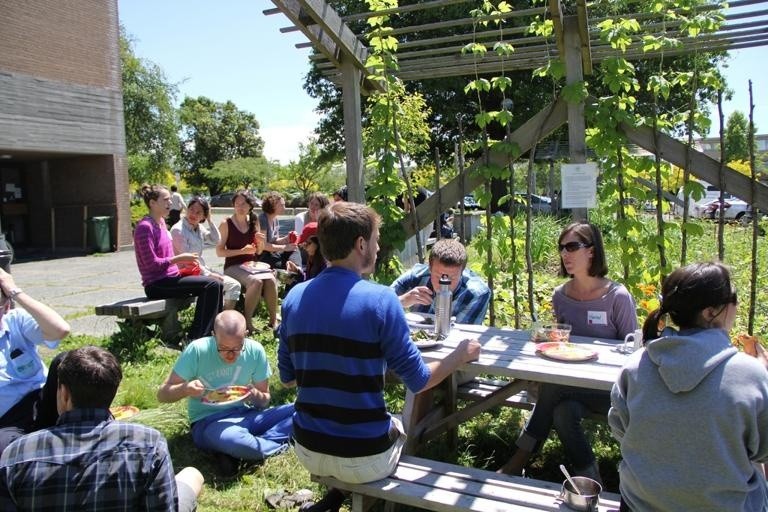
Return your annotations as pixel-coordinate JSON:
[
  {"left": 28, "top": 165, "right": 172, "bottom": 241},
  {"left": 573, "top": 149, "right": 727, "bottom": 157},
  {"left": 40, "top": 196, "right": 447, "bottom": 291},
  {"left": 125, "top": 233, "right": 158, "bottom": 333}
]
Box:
[
  {"left": 457, "top": 377, "right": 608, "bottom": 422},
  {"left": 95, "top": 297, "right": 196, "bottom": 340},
  {"left": 310, "top": 456, "right": 622, "bottom": 512}
]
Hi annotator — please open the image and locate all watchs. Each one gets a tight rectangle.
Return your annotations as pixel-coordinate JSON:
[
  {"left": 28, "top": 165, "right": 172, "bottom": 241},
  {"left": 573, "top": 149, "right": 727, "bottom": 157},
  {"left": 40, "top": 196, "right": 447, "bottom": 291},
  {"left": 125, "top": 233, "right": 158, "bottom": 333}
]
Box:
[{"left": 7, "top": 288, "right": 23, "bottom": 296}]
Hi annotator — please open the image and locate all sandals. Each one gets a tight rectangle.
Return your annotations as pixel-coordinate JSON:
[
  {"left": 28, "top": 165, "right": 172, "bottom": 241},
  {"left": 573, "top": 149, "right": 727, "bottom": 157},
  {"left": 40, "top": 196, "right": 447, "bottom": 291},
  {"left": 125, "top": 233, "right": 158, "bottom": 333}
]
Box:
[
  {"left": 270, "top": 318, "right": 280, "bottom": 328},
  {"left": 246, "top": 319, "right": 261, "bottom": 334}
]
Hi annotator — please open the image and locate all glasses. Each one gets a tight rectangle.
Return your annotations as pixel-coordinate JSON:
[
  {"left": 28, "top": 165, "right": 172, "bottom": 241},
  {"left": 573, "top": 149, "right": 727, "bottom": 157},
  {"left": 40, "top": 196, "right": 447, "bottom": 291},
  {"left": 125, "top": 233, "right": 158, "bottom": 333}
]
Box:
[
  {"left": 0, "top": 299, "right": 10, "bottom": 311},
  {"left": 706, "top": 291, "right": 739, "bottom": 308},
  {"left": 217, "top": 344, "right": 246, "bottom": 354},
  {"left": 558, "top": 241, "right": 591, "bottom": 252}
]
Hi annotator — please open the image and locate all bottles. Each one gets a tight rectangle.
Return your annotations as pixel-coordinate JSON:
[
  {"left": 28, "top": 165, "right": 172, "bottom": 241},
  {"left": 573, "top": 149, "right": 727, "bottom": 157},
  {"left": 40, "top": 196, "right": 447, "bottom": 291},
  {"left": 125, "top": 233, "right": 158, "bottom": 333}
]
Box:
[{"left": 434, "top": 273, "right": 453, "bottom": 335}]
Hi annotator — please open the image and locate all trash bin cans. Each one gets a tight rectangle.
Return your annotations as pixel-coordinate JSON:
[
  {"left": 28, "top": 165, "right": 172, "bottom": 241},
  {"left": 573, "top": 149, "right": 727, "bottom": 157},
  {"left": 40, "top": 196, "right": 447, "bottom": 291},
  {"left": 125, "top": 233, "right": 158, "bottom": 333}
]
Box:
[{"left": 90, "top": 216, "right": 115, "bottom": 252}]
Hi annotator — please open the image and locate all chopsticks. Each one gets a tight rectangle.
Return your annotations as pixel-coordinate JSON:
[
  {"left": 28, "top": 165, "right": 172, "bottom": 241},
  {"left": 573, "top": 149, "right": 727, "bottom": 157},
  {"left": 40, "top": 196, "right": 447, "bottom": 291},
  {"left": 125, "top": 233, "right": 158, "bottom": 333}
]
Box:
[{"left": 200, "top": 385, "right": 251, "bottom": 406}]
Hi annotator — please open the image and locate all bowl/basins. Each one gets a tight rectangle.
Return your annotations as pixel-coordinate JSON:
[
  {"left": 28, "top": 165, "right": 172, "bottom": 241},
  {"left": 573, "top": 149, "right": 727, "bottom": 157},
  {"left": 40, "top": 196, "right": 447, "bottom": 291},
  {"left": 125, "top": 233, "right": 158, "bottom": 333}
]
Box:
[
  {"left": 562, "top": 476, "right": 602, "bottom": 511},
  {"left": 544, "top": 323, "right": 572, "bottom": 343}
]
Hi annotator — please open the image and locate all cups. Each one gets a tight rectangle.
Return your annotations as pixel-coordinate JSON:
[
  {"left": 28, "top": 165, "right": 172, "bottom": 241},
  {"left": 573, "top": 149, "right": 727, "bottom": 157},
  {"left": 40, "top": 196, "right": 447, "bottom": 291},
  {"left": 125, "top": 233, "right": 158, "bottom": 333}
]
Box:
[
  {"left": 288, "top": 231, "right": 296, "bottom": 243},
  {"left": 624, "top": 329, "right": 643, "bottom": 352}
]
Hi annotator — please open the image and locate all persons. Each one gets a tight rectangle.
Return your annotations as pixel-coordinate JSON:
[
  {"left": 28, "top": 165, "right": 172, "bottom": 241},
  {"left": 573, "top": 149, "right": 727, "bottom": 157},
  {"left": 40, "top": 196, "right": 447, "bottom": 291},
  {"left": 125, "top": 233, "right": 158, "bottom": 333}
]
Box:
[
  {"left": 170, "top": 196, "right": 241, "bottom": 310},
  {"left": 135, "top": 185, "right": 223, "bottom": 336},
  {"left": 495, "top": 223, "right": 639, "bottom": 490},
  {"left": 390, "top": 238, "right": 492, "bottom": 385},
  {"left": 0, "top": 346, "right": 204, "bottom": 512},
  {"left": 287, "top": 222, "right": 328, "bottom": 280},
  {"left": 277, "top": 202, "right": 481, "bottom": 484},
  {"left": 157, "top": 310, "right": 295, "bottom": 477},
  {"left": 608, "top": 263, "right": 768, "bottom": 512},
  {"left": 216, "top": 191, "right": 278, "bottom": 335},
  {"left": 333, "top": 186, "right": 348, "bottom": 201},
  {"left": 168, "top": 185, "right": 187, "bottom": 228},
  {"left": 256, "top": 193, "right": 298, "bottom": 269},
  {"left": 1, "top": 267, "right": 69, "bottom": 455},
  {"left": 288, "top": 192, "right": 330, "bottom": 265}
]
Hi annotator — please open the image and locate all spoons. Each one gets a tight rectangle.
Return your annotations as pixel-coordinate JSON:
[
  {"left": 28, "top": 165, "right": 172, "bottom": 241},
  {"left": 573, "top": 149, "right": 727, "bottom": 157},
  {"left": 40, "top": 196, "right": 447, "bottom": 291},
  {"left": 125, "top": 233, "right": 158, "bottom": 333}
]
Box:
[{"left": 204, "top": 387, "right": 226, "bottom": 394}]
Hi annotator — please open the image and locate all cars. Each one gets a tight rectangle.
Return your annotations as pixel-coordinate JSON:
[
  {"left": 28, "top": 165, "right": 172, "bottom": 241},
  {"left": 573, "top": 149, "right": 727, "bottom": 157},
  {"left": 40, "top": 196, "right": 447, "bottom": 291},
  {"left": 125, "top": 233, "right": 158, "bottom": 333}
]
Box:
[
  {"left": 715, "top": 200, "right": 752, "bottom": 221},
  {"left": 695, "top": 199, "right": 718, "bottom": 219},
  {"left": 210, "top": 192, "right": 262, "bottom": 207},
  {"left": 515, "top": 193, "right": 557, "bottom": 215}
]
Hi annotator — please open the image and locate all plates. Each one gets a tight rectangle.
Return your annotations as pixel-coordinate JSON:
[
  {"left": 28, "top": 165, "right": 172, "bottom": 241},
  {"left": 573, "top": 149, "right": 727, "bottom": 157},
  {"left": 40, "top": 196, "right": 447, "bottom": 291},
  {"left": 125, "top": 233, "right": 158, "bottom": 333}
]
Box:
[
  {"left": 404, "top": 312, "right": 457, "bottom": 329},
  {"left": 536, "top": 342, "right": 599, "bottom": 361},
  {"left": 109, "top": 406, "right": 140, "bottom": 420},
  {"left": 244, "top": 261, "right": 270, "bottom": 271},
  {"left": 410, "top": 336, "right": 438, "bottom": 349}
]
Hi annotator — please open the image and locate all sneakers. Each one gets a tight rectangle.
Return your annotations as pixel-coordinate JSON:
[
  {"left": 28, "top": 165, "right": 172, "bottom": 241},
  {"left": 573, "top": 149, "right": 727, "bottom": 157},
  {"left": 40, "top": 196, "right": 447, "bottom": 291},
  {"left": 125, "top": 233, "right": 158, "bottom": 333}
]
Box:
[{"left": 214, "top": 451, "right": 240, "bottom": 476}]
